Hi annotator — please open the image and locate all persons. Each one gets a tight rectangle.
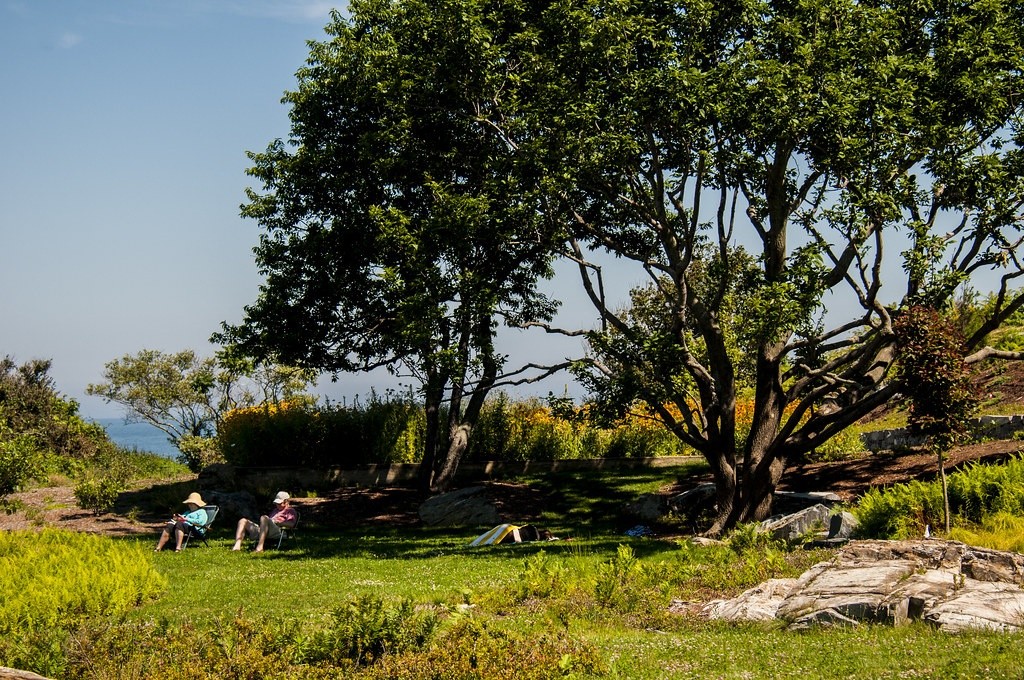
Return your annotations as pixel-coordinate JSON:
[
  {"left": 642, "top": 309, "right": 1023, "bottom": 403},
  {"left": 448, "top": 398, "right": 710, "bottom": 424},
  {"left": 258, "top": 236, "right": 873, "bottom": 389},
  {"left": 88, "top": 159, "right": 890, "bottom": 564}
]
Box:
[
  {"left": 152, "top": 493, "right": 209, "bottom": 554},
  {"left": 231, "top": 492, "right": 298, "bottom": 552}
]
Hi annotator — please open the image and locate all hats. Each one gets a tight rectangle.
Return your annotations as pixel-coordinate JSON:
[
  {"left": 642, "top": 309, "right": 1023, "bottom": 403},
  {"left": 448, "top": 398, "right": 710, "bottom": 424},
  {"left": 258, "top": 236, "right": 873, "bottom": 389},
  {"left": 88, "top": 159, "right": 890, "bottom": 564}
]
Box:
[
  {"left": 182, "top": 492, "right": 206, "bottom": 507},
  {"left": 273, "top": 491, "right": 290, "bottom": 503}
]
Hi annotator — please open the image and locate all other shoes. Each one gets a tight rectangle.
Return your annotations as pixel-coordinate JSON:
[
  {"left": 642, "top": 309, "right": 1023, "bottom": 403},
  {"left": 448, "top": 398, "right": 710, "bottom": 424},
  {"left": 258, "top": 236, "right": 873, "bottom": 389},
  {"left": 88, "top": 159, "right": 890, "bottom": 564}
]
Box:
[{"left": 250, "top": 549, "right": 264, "bottom": 554}]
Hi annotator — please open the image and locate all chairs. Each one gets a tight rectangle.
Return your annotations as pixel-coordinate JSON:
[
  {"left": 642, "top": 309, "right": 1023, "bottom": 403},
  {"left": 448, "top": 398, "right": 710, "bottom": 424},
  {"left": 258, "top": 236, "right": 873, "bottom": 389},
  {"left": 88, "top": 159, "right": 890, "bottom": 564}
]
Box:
[
  {"left": 182, "top": 505, "right": 220, "bottom": 550},
  {"left": 253, "top": 512, "right": 301, "bottom": 551}
]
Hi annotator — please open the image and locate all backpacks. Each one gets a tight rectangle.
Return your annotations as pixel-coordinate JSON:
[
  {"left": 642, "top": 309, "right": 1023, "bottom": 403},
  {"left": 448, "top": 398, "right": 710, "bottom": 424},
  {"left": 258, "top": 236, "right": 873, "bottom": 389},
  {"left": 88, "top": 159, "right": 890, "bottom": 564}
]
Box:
[{"left": 624, "top": 525, "right": 654, "bottom": 537}]
